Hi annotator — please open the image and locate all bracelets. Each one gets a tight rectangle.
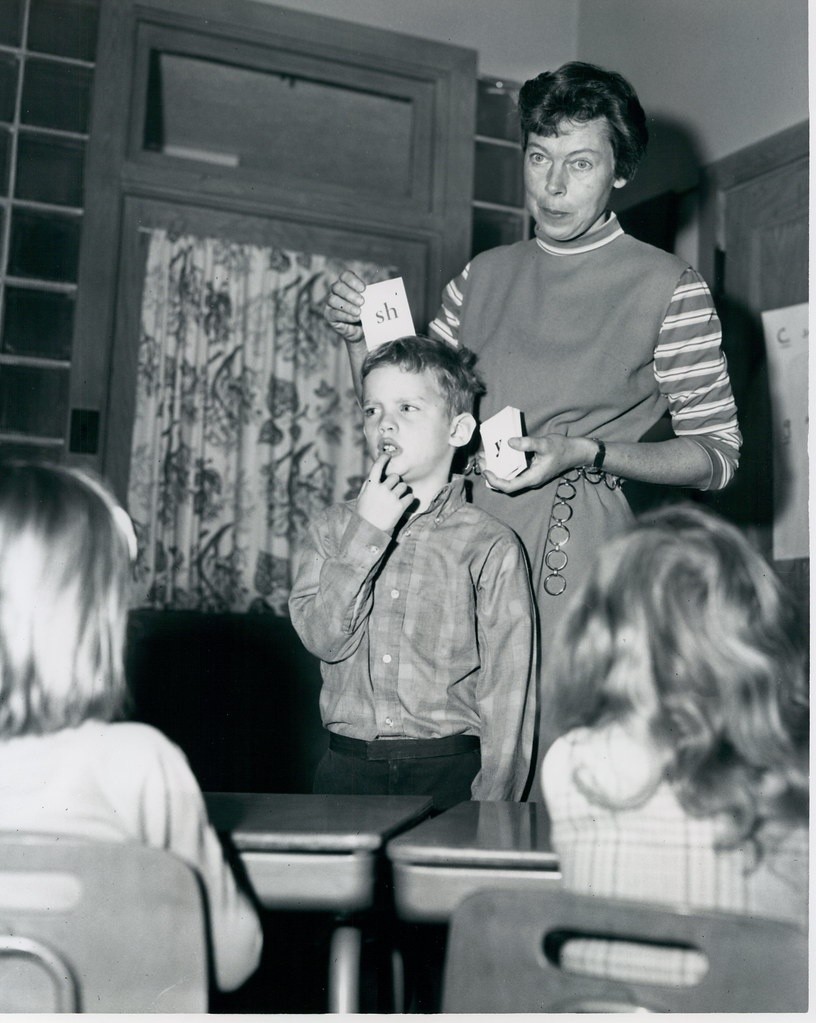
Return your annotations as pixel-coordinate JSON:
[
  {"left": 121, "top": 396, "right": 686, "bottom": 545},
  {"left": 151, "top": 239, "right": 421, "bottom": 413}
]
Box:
[{"left": 586, "top": 438, "right": 605, "bottom": 473}]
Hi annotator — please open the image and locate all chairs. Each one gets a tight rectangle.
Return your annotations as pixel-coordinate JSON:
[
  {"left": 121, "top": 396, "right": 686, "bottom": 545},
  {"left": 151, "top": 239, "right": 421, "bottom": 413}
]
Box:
[
  {"left": 0, "top": 829, "right": 209, "bottom": 1014},
  {"left": 442, "top": 883, "right": 809, "bottom": 1013}
]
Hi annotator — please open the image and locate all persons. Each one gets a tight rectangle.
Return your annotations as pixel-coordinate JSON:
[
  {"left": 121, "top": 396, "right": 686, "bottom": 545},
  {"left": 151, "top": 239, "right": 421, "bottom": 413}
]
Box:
[
  {"left": 288, "top": 337, "right": 538, "bottom": 1013},
  {"left": 1, "top": 459, "right": 262, "bottom": 991},
  {"left": 327, "top": 60, "right": 744, "bottom": 801},
  {"left": 539, "top": 503, "right": 810, "bottom": 985}
]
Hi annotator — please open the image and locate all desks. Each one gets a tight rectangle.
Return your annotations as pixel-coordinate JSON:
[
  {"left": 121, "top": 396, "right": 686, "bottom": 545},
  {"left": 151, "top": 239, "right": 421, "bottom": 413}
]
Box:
[
  {"left": 389, "top": 801, "right": 563, "bottom": 1014},
  {"left": 199, "top": 791, "right": 435, "bottom": 1014}
]
